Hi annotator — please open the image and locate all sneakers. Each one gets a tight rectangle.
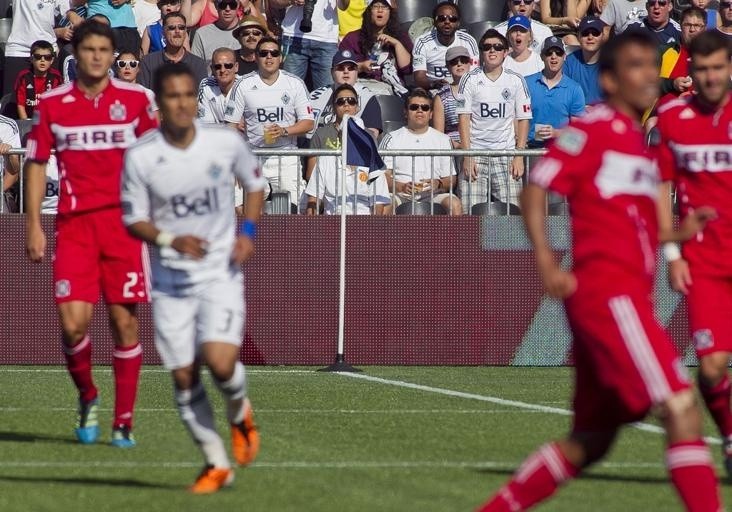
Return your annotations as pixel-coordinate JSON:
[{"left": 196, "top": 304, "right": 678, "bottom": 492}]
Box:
[
  {"left": 188, "top": 463, "right": 235, "bottom": 494},
  {"left": 75, "top": 395, "right": 99, "bottom": 444},
  {"left": 111, "top": 425, "right": 136, "bottom": 448},
  {"left": 230, "top": 397, "right": 259, "bottom": 467}
]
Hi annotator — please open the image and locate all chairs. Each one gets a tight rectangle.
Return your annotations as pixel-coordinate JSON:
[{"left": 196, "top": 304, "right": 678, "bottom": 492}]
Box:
[{"left": 389, "top": 0, "right": 511, "bottom": 54}]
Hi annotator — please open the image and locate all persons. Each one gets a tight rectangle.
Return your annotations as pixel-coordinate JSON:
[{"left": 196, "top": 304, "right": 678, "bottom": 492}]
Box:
[
  {"left": 21, "top": 16, "right": 159, "bottom": 448},
  {"left": 652, "top": 24, "right": 731, "bottom": 484},
  {"left": 117, "top": 56, "right": 271, "bottom": 498},
  {"left": 1, "top": 2, "right": 730, "bottom": 218},
  {"left": 473, "top": 27, "right": 722, "bottom": 512}
]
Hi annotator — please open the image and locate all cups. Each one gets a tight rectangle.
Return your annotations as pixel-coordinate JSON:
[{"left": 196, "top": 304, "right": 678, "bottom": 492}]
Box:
[
  {"left": 264, "top": 123, "right": 277, "bottom": 145},
  {"left": 534, "top": 123, "right": 546, "bottom": 142},
  {"left": 414, "top": 177, "right": 424, "bottom": 193}
]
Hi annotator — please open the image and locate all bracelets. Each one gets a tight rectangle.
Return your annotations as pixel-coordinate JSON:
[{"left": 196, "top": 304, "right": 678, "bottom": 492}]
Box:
[
  {"left": 662, "top": 241, "right": 682, "bottom": 263},
  {"left": 156, "top": 229, "right": 176, "bottom": 249},
  {"left": 239, "top": 219, "right": 258, "bottom": 239}
]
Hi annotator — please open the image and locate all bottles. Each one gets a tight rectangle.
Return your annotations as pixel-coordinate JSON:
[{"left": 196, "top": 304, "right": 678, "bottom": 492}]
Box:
[{"left": 368, "top": 40, "right": 383, "bottom": 66}]
